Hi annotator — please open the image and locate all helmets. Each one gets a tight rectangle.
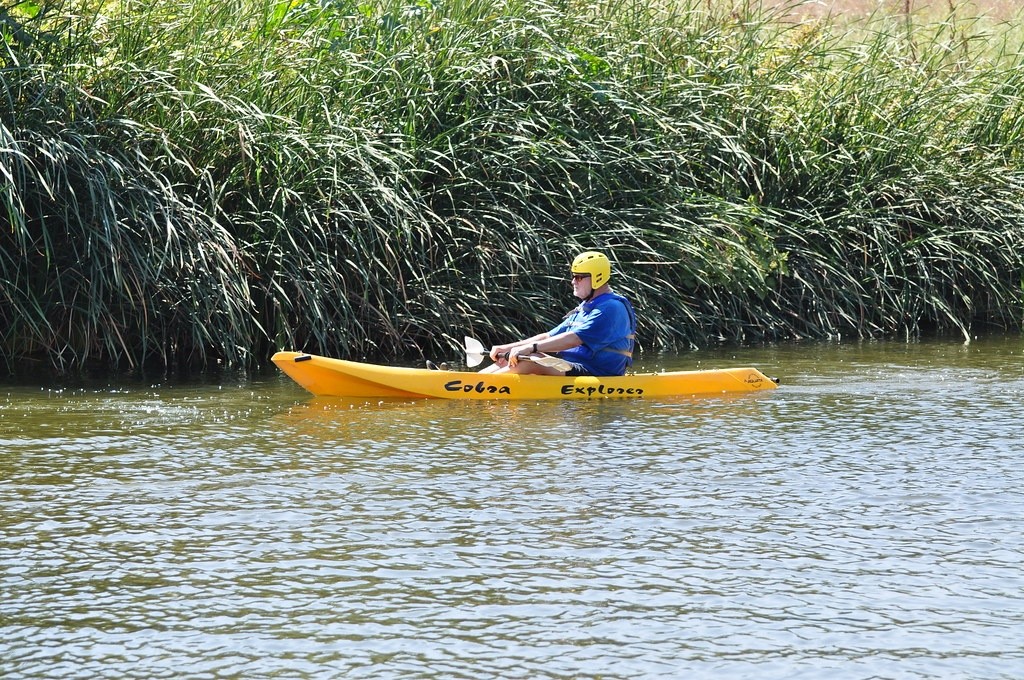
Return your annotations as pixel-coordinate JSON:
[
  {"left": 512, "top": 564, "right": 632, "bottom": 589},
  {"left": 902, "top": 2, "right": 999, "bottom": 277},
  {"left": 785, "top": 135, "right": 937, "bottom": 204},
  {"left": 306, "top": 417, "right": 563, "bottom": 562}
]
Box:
[{"left": 571, "top": 251, "right": 611, "bottom": 290}]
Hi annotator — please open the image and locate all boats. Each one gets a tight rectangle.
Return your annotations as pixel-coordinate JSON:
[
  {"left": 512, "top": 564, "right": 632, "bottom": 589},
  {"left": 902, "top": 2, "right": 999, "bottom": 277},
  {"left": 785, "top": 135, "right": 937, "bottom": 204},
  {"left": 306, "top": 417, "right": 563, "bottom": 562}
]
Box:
[{"left": 271, "top": 351, "right": 777, "bottom": 400}]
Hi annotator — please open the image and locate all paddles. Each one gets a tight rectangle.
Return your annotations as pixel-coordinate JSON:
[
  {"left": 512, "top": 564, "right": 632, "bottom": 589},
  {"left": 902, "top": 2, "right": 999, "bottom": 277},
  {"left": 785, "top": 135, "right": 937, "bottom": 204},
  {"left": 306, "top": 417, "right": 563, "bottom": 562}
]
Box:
[{"left": 464, "top": 335, "right": 573, "bottom": 375}]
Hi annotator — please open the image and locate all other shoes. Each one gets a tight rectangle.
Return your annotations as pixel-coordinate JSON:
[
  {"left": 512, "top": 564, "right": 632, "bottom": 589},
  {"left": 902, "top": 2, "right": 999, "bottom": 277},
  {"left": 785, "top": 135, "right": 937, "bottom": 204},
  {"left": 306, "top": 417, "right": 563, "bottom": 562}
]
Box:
[
  {"left": 440, "top": 362, "right": 449, "bottom": 371},
  {"left": 426, "top": 360, "right": 439, "bottom": 370}
]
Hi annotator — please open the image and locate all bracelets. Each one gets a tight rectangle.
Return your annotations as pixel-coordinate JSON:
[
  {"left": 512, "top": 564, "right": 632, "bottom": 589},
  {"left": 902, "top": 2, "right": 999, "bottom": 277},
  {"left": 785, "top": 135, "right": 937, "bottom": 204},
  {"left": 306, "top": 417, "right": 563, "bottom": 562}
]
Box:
[{"left": 532, "top": 344, "right": 537, "bottom": 353}]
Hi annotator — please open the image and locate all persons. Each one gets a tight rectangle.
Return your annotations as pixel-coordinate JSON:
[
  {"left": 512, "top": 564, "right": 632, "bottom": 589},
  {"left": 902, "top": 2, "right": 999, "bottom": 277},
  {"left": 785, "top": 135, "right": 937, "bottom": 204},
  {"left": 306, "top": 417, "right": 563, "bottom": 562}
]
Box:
[{"left": 426, "top": 251, "right": 636, "bottom": 377}]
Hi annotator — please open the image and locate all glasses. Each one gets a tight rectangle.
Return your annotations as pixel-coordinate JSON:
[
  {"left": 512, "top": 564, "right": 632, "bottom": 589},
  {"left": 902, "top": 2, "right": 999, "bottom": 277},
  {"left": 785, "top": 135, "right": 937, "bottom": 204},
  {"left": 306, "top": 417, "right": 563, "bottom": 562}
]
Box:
[{"left": 572, "top": 274, "right": 591, "bottom": 281}]
